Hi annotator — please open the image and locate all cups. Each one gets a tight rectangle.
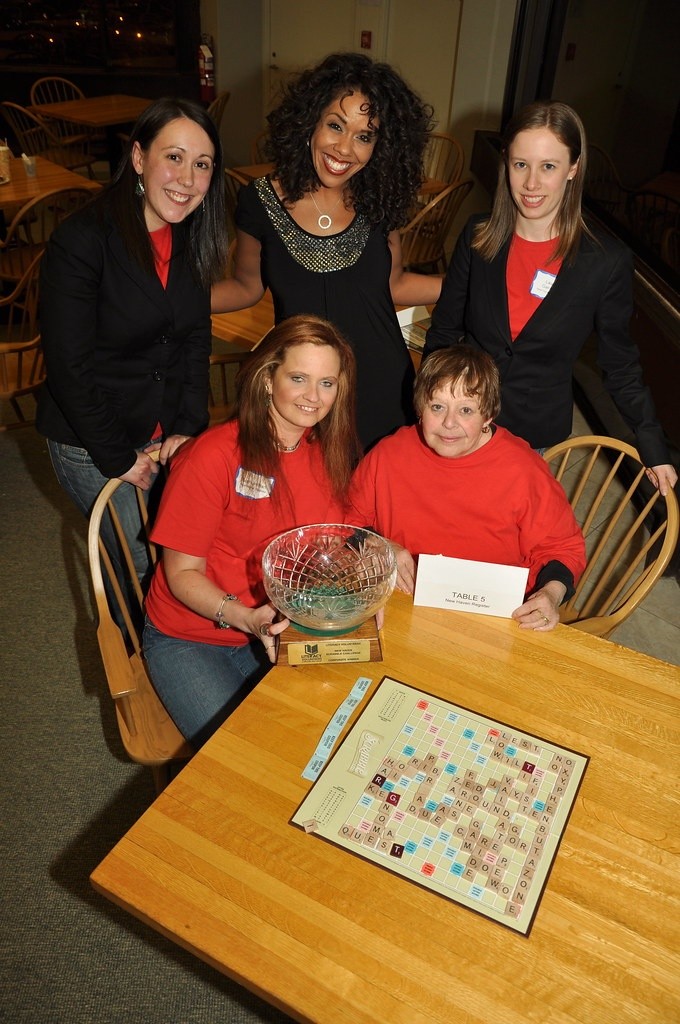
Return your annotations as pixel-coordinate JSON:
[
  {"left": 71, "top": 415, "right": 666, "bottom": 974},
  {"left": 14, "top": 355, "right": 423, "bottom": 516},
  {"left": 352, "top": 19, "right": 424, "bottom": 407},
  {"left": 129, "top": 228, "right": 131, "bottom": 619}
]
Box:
[
  {"left": 0, "top": 147, "right": 11, "bottom": 184},
  {"left": 23, "top": 156, "right": 36, "bottom": 177}
]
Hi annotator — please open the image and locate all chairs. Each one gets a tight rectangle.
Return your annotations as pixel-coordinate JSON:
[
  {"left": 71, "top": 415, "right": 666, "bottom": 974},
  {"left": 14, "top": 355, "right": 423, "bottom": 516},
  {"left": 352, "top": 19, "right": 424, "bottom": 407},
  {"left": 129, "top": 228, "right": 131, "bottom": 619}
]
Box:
[
  {"left": 403, "top": 131, "right": 465, "bottom": 271},
  {"left": 0, "top": 101, "right": 97, "bottom": 180},
  {"left": 583, "top": 142, "right": 680, "bottom": 274},
  {"left": 544, "top": 435, "right": 680, "bottom": 640},
  {"left": 208, "top": 351, "right": 248, "bottom": 424},
  {"left": 86, "top": 448, "right": 197, "bottom": 799},
  {"left": 222, "top": 168, "right": 250, "bottom": 280},
  {"left": 30, "top": 77, "right": 88, "bottom": 146},
  {"left": 251, "top": 131, "right": 271, "bottom": 163},
  {"left": 400, "top": 178, "right": 474, "bottom": 274},
  {"left": 0, "top": 248, "right": 47, "bottom": 433},
  {"left": 207, "top": 90, "right": 230, "bottom": 127},
  {"left": 0, "top": 187, "right": 96, "bottom": 295}
]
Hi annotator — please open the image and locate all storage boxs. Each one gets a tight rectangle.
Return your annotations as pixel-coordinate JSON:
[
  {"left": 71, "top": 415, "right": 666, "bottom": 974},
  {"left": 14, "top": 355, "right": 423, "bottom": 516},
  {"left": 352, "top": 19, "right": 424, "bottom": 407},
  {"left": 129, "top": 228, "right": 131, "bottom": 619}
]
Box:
[{"left": 274, "top": 611, "right": 384, "bottom": 665}]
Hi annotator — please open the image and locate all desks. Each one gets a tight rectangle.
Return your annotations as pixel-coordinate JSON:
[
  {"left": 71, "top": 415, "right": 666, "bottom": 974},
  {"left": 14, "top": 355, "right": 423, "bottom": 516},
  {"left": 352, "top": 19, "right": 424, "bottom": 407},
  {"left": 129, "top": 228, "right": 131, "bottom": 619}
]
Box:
[
  {"left": 87, "top": 585, "right": 680, "bottom": 1024},
  {"left": 0, "top": 155, "right": 104, "bottom": 224},
  {"left": 26, "top": 95, "right": 154, "bottom": 178},
  {"left": 232, "top": 161, "right": 448, "bottom": 194},
  {"left": 634, "top": 171, "right": 680, "bottom": 214}
]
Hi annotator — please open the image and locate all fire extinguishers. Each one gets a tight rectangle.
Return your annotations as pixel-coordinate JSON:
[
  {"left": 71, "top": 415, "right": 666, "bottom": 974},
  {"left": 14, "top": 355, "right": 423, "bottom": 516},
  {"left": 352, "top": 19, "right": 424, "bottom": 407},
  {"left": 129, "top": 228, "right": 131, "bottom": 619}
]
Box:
[{"left": 198, "top": 34, "right": 216, "bottom": 102}]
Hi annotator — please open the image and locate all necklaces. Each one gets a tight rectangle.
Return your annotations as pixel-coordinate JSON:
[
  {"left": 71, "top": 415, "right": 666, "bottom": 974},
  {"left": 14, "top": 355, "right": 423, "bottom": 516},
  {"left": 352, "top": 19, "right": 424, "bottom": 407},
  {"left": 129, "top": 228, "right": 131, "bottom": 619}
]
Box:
[
  {"left": 273, "top": 434, "right": 304, "bottom": 450},
  {"left": 309, "top": 180, "right": 350, "bottom": 229}
]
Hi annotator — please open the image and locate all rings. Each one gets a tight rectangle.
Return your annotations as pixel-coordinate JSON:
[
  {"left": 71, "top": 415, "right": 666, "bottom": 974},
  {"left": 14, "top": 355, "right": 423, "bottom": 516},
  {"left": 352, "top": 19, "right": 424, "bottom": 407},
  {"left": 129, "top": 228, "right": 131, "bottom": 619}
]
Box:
[
  {"left": 266, "top": 645, "right": 276, "bottom": 654},
  {"left": 537, "top": 609, "right": 550, "bottom": 625},
  {"left": 260, "top": 622, "right": 273, "bottom": 637}
]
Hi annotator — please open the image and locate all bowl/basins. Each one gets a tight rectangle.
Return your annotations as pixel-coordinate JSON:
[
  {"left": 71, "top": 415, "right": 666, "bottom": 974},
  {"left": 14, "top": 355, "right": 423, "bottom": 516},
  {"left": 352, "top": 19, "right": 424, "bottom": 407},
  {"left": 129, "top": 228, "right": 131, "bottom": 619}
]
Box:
[{"left": 262, "top": 522, "right": 396, "bottom": 631}]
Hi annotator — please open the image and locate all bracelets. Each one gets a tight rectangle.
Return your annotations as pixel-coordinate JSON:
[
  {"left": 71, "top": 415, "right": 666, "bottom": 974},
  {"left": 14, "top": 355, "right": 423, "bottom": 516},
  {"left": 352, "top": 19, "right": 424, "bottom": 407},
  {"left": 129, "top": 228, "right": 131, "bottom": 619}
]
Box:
[{"left": 218, "top": 593, "right": 241, "bottom": 629}]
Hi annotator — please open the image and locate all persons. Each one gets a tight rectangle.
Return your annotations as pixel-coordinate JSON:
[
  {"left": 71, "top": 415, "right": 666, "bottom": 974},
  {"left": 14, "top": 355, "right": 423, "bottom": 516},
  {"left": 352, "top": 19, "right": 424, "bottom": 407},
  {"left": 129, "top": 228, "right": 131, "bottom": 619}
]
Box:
[
  {"left": 419, "top": 104, "right": 678, "bottom": 496},
  {"left": 144, "top": 315, "right": 364, "bottom": 753},
  {"left": 33, "top": 96, "right": 237, "bottom": 680},
  {"left": 211, "top": 52, "right": 445, "bottom": 470},
  {"left": 346, "top": 345, "right": 587, "bottom": 633}
]
